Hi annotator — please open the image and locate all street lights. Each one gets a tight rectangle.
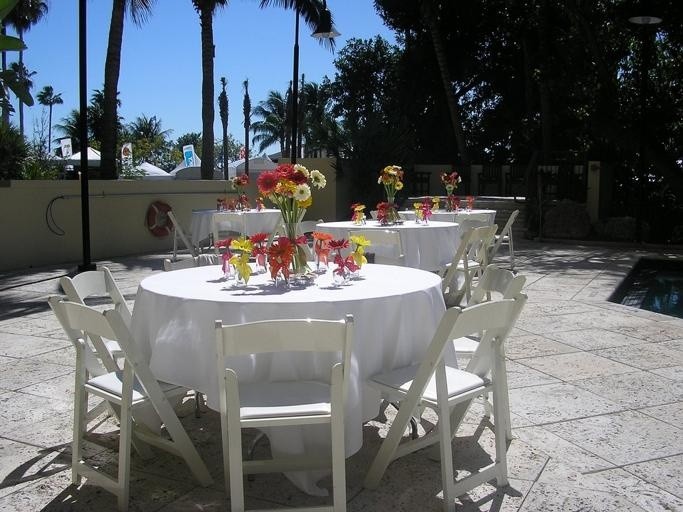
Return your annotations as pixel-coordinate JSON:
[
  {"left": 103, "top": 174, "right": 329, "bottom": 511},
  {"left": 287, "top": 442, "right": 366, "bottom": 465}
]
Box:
[{"left": 291, "top": 0, "right": 341, "bottom": 207}]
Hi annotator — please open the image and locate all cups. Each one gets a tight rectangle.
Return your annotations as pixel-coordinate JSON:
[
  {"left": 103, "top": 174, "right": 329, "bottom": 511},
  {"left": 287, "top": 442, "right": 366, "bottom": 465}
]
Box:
[
  {"left": 224, "top": 254, "right": 352, "bottom": 286},
  {"left": 355, "top": 215, "right": 430, "bottom": 226}
]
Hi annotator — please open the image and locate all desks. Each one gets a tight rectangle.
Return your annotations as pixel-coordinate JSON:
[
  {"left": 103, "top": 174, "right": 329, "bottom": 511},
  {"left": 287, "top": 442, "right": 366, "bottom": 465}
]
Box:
[
  {"left": 192, "top": 207, "right": 282, "bottom": 262},
  {"left": 129, "top": 261, "right": 458, "bottom": 484},
  {"left": 370, "top": 209, "right": 498, "bottom": 263},
  {"left": 315, "top": 219, "right": 461, "bottom": 297}
]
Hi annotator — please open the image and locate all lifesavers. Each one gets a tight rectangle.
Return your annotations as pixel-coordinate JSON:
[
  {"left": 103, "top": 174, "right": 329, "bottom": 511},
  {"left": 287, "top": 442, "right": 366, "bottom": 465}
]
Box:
[{"left": 147, "top": 202, "right": 174, "bottom": 236}]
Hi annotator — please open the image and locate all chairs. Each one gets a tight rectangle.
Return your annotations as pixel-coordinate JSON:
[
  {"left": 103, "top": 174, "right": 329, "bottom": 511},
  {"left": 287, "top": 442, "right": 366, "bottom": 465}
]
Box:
[
  {"left": 48, "top": 296, "right": 215, "bottom": 511},
  {"left": 420, "top": 265, "right": 526, "bottom": 444},
  {"left": 348, "top": 229, "right": 406, "bottom": 267},
  {"left": 362, "top": 292, "right": 529, "bottom": 511},
  {"left": 168, "top": 211, "right": 210, "bottom": 260},
  {"left": 208, "top": 314, "right": 355, "bottom": 512},
  {"left": 439, "top": 224, "right": 498, "bottom": 309},
  {"left": 488, "top": 210, "right": 520, "bottom": 269},
  {"left": 60, "top": 266, "right": 207, "bottom": 436}
]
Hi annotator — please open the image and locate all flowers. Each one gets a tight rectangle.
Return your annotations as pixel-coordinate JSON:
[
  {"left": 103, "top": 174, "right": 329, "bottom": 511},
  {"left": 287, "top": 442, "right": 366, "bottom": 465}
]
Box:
[
  {"left": 216, "top": 162, "right": 371, "bottom": 287},
  {"left": 351, "top": 164, "right": 475, "bottom": 227}
]
